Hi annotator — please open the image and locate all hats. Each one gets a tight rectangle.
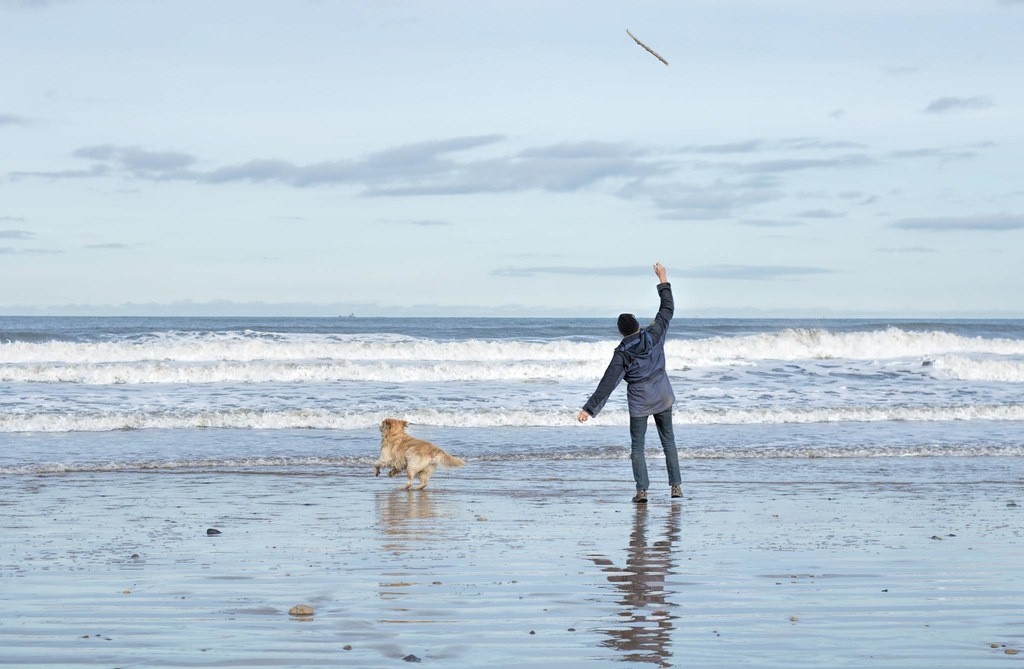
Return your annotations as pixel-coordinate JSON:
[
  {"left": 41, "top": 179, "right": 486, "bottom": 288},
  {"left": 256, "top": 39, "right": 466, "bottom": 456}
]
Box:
[{"left": 617, "top": 314, "right": 639, "bottom": 336}]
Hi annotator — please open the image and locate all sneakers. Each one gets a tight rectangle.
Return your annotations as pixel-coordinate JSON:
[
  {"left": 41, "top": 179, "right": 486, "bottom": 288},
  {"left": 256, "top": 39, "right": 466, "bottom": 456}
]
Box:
[
  {"left": 633, "top": 491, "right": 647, "bottom": 502},
  {"left": 671, "top": 486, "right": 683, "bottom": 498}
]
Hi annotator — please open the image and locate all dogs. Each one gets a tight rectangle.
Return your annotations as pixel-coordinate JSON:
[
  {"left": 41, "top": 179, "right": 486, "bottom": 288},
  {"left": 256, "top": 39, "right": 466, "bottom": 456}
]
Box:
[{"left": 374, "top": 419, "right": 466, "bottom": 491}]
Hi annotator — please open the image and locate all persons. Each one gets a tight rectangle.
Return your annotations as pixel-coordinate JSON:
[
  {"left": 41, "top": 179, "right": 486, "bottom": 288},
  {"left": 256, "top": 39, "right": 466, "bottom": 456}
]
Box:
[{"left": 578, "top": 263, "right": 683, "bottom": 502}]
